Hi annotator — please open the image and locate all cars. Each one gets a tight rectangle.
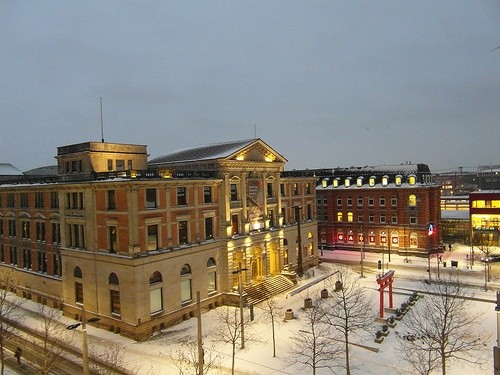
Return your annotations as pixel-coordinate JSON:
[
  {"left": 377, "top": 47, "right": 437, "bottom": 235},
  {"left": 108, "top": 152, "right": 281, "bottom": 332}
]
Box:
[{"left": 481, "top": 253, "right": 500, "bottom": 263}]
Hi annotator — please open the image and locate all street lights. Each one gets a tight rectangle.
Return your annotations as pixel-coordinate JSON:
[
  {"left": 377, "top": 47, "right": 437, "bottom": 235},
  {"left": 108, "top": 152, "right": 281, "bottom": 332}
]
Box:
[
  {"left": 232, "top": 261, "right": 248, "bottom": 348},
  {"left": 66, "top": 307, "right": 100, "bottom": 375}
]
